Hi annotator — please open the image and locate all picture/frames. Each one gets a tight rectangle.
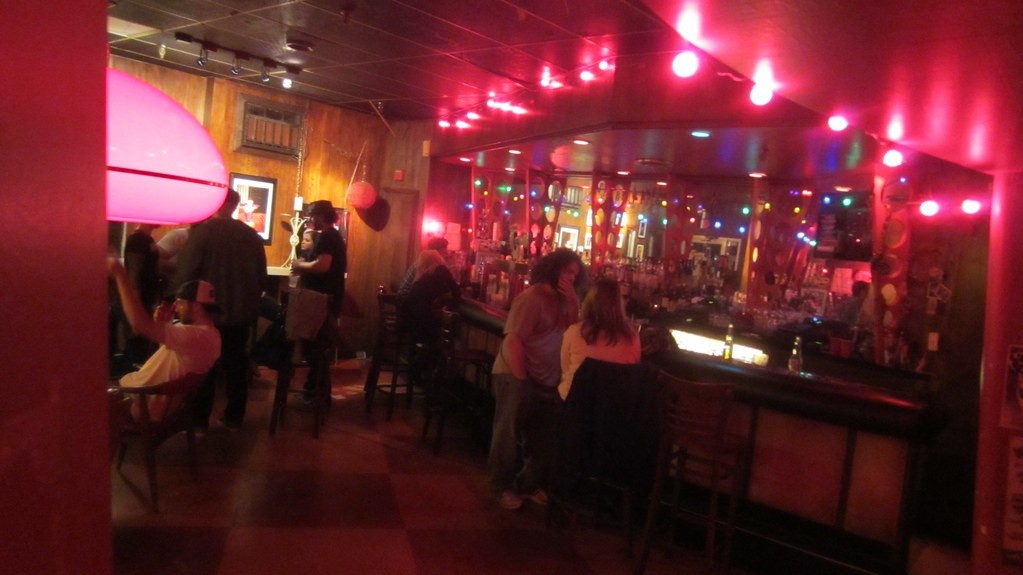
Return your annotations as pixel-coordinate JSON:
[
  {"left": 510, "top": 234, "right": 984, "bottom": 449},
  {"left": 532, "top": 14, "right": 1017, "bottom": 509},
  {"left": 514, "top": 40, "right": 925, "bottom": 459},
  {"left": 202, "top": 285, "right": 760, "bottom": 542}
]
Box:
[{"left": 227, "top": 172, "right": 278, "bottom": 245}]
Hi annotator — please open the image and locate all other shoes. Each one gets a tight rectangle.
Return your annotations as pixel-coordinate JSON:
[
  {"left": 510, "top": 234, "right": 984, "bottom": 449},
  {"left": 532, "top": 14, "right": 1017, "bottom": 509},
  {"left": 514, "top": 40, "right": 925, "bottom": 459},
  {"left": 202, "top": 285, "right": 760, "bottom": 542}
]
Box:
[
  {"left": 494, "top": 488, "right": 523, "bottom": 510},
  {"left": 303, "top": 385, "right": 313, "bottom": 404},
  {"left": 298, "top": 400, "right": 331, "bottom": 412},
  {"left": 528, "top": 489, "right": 552, "bottom": 507},
  {"left": 209, "top": 416, "right": 240, "bottom": 432},
  {"left": 193, "top": 420, "right": 209, "bottom": 437}
]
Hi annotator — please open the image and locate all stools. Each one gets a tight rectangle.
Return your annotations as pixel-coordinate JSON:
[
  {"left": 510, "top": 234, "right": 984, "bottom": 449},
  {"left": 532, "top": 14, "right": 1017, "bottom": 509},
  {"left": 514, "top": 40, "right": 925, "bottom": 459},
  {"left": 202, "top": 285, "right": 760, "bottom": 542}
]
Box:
[{"left": 362, "top": 284, "right": 751, "bottom": 575}]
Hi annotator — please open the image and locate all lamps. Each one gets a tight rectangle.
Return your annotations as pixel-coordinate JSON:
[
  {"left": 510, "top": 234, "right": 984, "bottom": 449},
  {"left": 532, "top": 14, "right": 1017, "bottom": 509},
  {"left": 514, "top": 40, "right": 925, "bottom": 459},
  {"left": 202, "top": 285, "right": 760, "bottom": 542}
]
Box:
[
  {"left": 281, "top": 66, "right": 300, "bottom": 88},
  {"left": 195, "top": 45, "right": 210, "bottom": 66},
  {"left": 231, "top": 53, "right": 249, "bottom": 75},
  {"left": 261, "top": 62, "right": 277, "bottom": 82}
]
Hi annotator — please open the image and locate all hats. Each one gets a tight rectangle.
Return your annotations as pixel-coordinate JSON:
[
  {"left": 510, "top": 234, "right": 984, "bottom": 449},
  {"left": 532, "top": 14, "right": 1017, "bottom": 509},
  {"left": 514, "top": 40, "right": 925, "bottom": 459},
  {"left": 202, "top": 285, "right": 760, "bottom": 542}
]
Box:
[
  {"left": 176, "top": 279, "right": 217, "bottom": 303},
  {"left": 428, "top": 237, "right": 448, "bottom": 249},
  {"left": 308, "top": 200, "right": 336, "bottom": 217}
]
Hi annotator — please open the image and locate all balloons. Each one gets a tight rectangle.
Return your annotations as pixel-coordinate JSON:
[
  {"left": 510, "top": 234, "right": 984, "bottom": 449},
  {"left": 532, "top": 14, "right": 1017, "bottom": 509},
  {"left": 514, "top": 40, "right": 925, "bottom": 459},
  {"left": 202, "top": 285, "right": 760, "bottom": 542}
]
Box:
[{"left": 349, "top": 181, "right": 377, "bottom": 210}]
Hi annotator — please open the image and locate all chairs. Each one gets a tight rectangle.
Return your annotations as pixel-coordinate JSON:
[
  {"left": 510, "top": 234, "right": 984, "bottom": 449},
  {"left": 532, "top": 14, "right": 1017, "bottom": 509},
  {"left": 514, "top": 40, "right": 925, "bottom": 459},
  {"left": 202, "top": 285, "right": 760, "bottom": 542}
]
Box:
[
  {"left": 268, "top": 324, "right": 336, "bottom": 438},
  {"left": 107, "top": 371, "right": 206, "bottom": 514}
]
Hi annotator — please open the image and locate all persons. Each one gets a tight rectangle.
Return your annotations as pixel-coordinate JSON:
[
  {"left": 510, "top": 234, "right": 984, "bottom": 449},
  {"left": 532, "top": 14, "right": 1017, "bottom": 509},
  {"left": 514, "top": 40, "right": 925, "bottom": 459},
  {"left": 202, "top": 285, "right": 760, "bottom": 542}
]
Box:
[
  {"left": 555, "top": 277, "right": 642, "bottom": 400},
  {"left": 492, "top": 247, "right": 590, "bottom": 508},
  {"left": 291, "top": 201, "right": 347, "bottom": 410},
  {"left": 625, "top": 297, "right": 683, "bottom": 365},
  {"left": 106, "top": 189, "right": 267, "bottom": 439},
  {"left": 838, "top": 279, "right": 872, "bottom": 327},
  {"left": 398, "top": 236, "right": 462, "bottom": 366}
]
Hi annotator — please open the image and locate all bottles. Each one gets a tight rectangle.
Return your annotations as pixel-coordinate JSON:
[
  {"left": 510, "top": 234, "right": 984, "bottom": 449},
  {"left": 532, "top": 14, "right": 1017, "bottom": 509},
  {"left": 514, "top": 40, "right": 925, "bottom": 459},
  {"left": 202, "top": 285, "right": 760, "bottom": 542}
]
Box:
[
  {"left": 444, "top": 230, "right": 860, "bottom": 356},
  {"left": 722, "top": 323, "right": 734, "bottom": 360},
  {"left": 788, "top": 335, "right": 803, "bottom": 374}
]
